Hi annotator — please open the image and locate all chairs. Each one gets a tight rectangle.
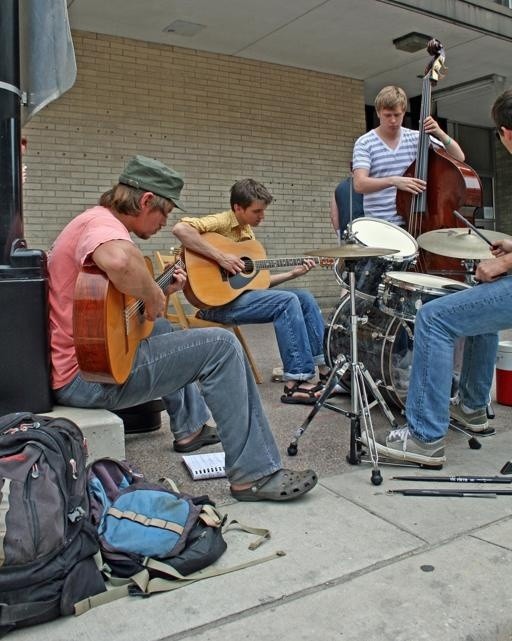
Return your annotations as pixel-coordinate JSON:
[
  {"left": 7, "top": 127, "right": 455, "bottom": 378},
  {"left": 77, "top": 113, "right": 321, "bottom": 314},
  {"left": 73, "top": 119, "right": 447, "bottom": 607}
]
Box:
[{"left": 151, "top": 246, "right": 264, "bottom": 389}]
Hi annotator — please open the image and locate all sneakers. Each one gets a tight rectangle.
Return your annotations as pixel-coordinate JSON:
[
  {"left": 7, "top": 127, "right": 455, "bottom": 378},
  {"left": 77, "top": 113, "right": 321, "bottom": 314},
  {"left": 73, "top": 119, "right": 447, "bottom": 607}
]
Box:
[
  {"left": 448, "top": 394, "right": 490, "bottom": 432},
  {"left": 359, "top": 422, "right": 447, "bottom": 468}
]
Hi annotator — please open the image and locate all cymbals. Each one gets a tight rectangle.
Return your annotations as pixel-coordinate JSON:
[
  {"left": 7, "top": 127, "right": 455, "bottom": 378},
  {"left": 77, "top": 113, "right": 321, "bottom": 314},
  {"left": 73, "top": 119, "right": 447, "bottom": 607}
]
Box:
[{"left": 417, "top": 228, "right": 512, "bottom": 260}]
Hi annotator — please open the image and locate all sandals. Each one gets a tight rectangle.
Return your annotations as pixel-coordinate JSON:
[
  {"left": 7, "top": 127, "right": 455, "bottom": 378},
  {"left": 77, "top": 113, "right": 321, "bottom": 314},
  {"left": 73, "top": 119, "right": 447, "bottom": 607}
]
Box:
[{"left": 280, "top": 367, "right": 334, "bottom": 405}]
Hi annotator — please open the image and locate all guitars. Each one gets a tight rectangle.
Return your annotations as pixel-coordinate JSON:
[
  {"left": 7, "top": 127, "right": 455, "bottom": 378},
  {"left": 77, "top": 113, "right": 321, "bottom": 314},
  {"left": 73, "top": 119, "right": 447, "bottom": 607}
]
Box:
[
  {"left": 178, "top": 232, "right": 336, "bottom": 309},
  {"left": 74, "top": 248, "right": 185, "bottom": 386}
]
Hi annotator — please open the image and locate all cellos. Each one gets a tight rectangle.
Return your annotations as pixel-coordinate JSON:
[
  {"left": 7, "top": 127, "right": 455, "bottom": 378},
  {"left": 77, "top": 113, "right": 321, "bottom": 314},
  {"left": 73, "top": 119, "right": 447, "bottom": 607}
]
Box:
[{"left": 396, "top": 38, "right": 482, "bottom": 288}]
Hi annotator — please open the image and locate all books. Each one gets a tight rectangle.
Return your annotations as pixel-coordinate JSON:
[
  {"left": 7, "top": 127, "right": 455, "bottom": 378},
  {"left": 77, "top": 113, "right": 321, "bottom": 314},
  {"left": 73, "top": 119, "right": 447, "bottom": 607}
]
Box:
[{"left": 181, "top": 452, "right": 226, "bottom": 480}]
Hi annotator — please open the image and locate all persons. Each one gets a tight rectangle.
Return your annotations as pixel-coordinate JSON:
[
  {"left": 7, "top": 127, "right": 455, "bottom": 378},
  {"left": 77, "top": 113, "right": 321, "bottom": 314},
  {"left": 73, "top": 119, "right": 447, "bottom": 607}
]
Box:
[
  {"left": 352, "top": 85, "right": 465, "bottom": 233},
  {"left": 362, "top": 88, "right": 512, "bottom": 467},
  {"left": 171, "top": 178, "right": 333, "bottom": 405},
  {"left": 46, "top": 154, "right": 318, "bottom": 502},
  {"left": 330, "top": 161, "right": 364, "bottom": 246}
]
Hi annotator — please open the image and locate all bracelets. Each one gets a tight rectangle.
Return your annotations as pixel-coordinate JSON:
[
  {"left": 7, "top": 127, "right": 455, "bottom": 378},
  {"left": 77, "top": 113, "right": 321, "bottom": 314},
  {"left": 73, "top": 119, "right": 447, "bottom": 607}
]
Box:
[{"left": 444, "top": 136, "right": 451, "bottom": 145}]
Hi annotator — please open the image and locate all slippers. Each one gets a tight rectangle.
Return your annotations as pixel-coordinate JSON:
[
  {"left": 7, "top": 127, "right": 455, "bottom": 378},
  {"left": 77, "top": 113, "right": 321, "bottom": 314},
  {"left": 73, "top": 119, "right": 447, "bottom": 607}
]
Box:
[
  {"left": 230, "top": 468, "right": 319, "bottom": 502},
  {"left": 173, "top": 423, "right": 222, "bottom": 454}
]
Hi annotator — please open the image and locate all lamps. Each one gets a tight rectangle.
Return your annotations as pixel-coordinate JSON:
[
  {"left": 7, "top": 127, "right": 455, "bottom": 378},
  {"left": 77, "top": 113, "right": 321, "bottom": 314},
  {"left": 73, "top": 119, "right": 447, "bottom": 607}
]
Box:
[
  {"left": 429, "top": 73, "right": 508, "bottom": 103},
  {"left": 392, "top": 31, "right": 434, "bottom": 54}
]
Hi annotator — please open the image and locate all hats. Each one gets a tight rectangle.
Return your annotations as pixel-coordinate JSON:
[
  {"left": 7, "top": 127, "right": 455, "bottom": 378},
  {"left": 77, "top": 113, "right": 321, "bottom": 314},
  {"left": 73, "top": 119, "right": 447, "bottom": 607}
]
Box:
[{"left": 115, "top": 154, "right": 190, "bottom": 214}]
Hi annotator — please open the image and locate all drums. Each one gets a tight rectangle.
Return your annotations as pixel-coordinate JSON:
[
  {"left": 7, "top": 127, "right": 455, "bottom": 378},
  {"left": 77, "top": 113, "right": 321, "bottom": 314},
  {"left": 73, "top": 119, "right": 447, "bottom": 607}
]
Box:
[
  {"left": 326, "top": 295, "right": 465, "bottom": 414},
  {"left": 376, "top": 272, "right": 473, "bottom": 324},
  {"left": 335, "top": 217, "right": 418, "bottom": 303}
]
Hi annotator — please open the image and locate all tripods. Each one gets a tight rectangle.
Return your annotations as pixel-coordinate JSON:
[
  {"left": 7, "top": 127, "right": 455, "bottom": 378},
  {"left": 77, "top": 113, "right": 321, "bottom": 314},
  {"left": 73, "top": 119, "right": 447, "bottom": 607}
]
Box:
[{"left": 288, "top": 262, "right": 398, "bottom": 485}]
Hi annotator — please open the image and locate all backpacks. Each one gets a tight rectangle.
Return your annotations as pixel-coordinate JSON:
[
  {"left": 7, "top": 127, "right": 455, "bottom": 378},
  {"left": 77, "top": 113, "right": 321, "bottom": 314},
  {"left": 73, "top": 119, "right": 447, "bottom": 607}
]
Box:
[
  {"left": 72, "top": 455, "right": 286, "bottom": 619},
  {"left": 0, "top": 409, "right": 108, "bottom": 631}
]
